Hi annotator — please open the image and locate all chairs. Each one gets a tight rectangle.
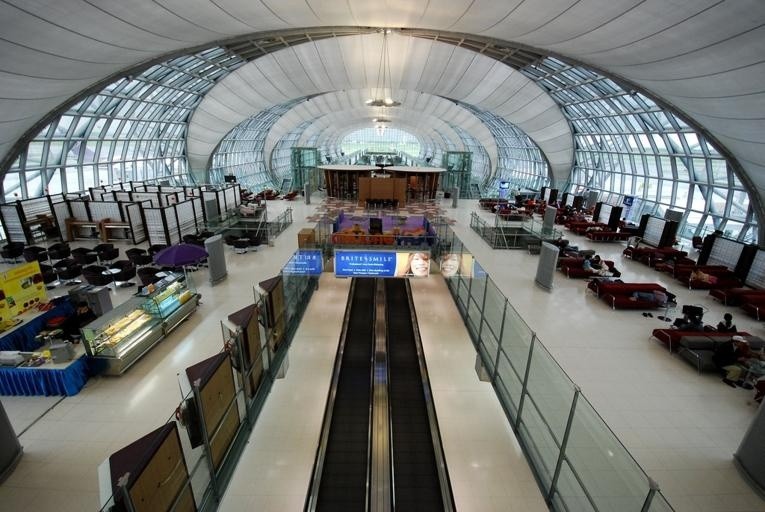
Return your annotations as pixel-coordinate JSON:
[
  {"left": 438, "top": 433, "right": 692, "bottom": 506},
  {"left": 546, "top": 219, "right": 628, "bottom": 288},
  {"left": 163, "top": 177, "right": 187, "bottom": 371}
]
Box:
[
  {"left": 240, "top": 185, "right": 299, "bottom": 215},
  {"left": 365, "top": 197, "right": 401, "bottom": 213},
  {"left": 0, "top": 230, "right": 265, "bottom": 294}
]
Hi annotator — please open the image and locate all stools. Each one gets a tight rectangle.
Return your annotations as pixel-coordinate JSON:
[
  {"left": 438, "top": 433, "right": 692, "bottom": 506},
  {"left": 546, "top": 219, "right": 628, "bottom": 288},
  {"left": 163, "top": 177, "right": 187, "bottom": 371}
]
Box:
[{"left": 45, "top": 316, "right": 67, "bottom": 329}]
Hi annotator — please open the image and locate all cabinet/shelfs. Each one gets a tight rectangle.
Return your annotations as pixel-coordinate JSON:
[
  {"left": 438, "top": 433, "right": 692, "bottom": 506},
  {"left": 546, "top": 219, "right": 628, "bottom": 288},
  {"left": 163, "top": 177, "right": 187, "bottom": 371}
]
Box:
[
  {"left": 141, "top": 272, "right": 201, "bottom": 338},
  {"left": 297, "top": 228, "right": 316, "bottom": 249},
  {"left": 80, "top": 297, "right": 165, "bottom": 378}
]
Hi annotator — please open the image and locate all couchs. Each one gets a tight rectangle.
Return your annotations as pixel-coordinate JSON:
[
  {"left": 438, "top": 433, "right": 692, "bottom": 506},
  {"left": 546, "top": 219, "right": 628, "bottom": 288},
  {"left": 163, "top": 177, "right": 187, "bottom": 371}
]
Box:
[
  {"left": 586, "top": 278, "right": 678, "bottom": 320},
  {"left": 478, "top": 191, "right": 638, "bottom": 242},
  {"left": 648, "top": 327, "right": 764, "bottom": 406},
  {"left": 621, "top": 245, "right": 764, "bottom": 323},
  {"left": 527, "top": 238, "right": 621, "bottom": 278}
]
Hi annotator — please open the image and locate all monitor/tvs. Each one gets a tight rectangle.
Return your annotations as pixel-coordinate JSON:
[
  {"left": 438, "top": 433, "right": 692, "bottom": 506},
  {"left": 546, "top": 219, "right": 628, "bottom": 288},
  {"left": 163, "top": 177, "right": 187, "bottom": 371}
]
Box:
[
  {"left": 500, "top": 182, "right": 509, "bottom": 189},
  {"left": 623, "top": 195, "right": 633, "bottom": 206},
  {"left": 224, "top": 175, "right": 236, "bottom": 182}
]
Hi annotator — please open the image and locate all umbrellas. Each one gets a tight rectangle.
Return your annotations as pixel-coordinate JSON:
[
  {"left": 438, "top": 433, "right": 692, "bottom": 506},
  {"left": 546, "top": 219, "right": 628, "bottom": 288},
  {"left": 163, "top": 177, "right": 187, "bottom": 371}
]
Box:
[{"left": 151, "top": 241, "right": 210, "bottom": 289}]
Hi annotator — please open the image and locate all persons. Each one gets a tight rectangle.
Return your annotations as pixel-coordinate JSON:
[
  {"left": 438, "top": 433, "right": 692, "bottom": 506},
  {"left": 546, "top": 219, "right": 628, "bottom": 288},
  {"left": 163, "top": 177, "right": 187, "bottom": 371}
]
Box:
[
  {"left": 492, "top": 187, "right": 627, "bottom": 234},
  {"left": 689, "top": 266, "right": 718, "bottom": 285},
  {"left": 584, "top": 253, "right": 615, "bottom": 278},
  {"left": 74, "top": 301, "right": 96, "bottom": 335},
  {"left": 440, "top": 254, "right": 466, "bottom": 278},
  {"left": 554, "top": 237, "right": 570, "bottom": 258},
  {"left": 678, "top": 309, "right": 765, "bottom": 403},
  {"left": 397, "top": 252, "right": 431, "bottom": 277}
]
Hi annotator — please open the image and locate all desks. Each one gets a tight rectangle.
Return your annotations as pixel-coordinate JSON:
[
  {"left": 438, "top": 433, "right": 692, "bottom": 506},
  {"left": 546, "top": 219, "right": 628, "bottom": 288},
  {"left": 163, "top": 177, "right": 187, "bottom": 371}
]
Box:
[
  {"left": 0, "top": 300, "right": 67, "bottom": 354},
  {"left": 98, "top": 218, "right": 131, "bottom": 242},
  {"left": 0, "top": 349, "right": 92, "bottom": 398},
  {"left": 66, "top": 215, "right": 101, "bottom": 243},
  {"left": 23, "top": 214, "right": 54, "bottom": 242}
]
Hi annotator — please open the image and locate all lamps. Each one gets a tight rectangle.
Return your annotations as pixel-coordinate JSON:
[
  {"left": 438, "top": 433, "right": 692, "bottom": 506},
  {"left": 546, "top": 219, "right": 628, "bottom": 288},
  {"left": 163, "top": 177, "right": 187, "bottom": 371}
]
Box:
[
  {"left": 370, "top": 107, "right": 395, "bottom": 125},
  {"left": 362, "top": 27, "right": 402, "bottom": 109}
]
